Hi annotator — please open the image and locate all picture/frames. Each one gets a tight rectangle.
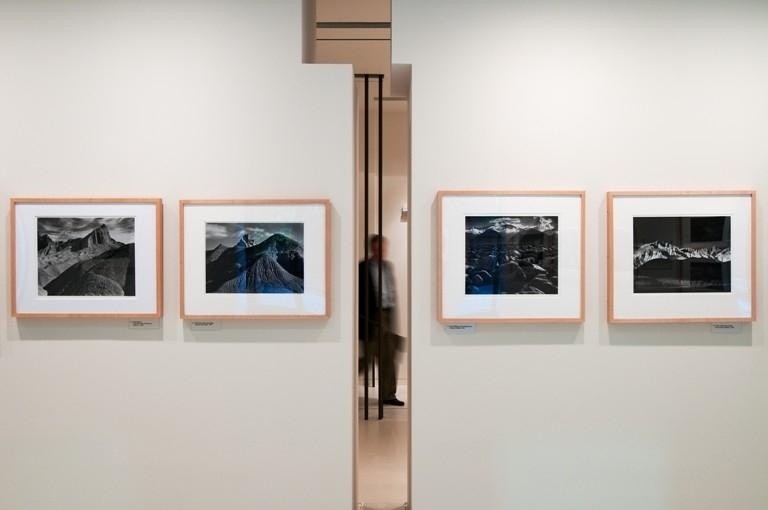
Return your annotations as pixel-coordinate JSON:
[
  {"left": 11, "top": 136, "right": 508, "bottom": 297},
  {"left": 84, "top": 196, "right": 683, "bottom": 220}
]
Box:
[
  {"left": 178, "top": 199, "right": 332, "bottom": 321},
  {"left": 608, "top": 190, "right": 757, "bottom": 324},
  {"left": 436, "top": 190, "right": 585, "bottom": 323},
  {"left": 9, "top": 198, "right": 163, "bottom": 321}
]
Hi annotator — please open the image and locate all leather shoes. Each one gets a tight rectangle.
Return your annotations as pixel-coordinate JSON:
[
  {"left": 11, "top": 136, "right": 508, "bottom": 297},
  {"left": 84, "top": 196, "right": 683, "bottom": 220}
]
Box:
[{"left": 383, "top": 398, "right": 405, "bottom": 406}]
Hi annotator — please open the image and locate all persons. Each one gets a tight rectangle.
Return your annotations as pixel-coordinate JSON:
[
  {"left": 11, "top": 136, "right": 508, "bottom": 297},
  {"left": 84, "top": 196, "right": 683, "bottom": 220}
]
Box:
[{"left": 358, "top": 233, "right": 406, "bottom": 407}]
